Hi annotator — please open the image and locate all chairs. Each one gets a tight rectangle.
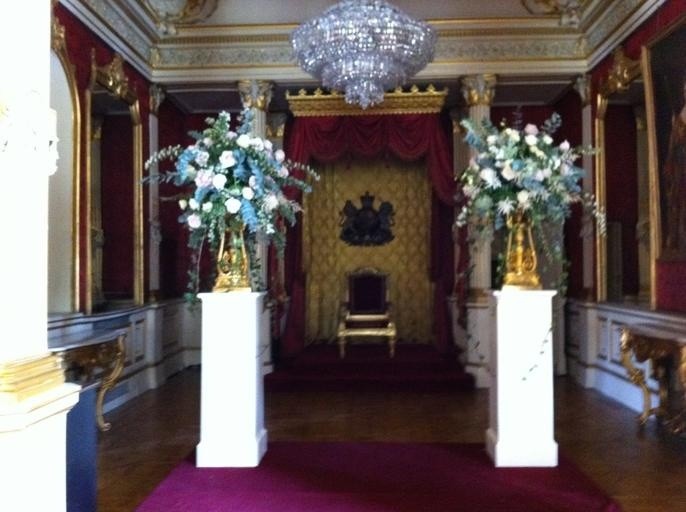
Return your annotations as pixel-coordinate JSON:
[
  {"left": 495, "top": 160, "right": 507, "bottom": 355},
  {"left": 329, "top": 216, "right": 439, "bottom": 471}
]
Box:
[{"left": 335, "top": 266, "right": 397, "bottom": 358}]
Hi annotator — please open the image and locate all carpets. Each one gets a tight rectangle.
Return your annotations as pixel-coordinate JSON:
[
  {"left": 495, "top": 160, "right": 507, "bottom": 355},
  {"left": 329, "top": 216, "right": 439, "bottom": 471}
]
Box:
[{"left": 130, "top": 441, "right": 627, "bottom": 511}]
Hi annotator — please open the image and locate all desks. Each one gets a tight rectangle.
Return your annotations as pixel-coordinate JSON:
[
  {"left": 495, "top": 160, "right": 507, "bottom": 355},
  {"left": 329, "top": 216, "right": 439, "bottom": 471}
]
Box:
[
  {"left": 616, "top": 323, "right": 684, "bottom": 439},
  {"left": 49, "top": 327, "right": 131, "bottom": 433}
]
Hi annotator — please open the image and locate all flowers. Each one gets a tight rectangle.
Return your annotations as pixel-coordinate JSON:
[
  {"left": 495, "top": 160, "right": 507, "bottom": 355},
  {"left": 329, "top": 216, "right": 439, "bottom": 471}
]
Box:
[
  {"left": 451, "top": 112, "right": 601, "bottom": 295},
  {"left": 140, "top": 109, "right": 321, "bottom": 316}
]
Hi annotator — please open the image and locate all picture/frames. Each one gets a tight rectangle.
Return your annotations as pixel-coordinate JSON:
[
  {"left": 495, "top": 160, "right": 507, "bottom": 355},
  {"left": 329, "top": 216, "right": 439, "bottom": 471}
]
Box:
[{"left": 642, "top": 12, "right": 686, "bottom": 263}]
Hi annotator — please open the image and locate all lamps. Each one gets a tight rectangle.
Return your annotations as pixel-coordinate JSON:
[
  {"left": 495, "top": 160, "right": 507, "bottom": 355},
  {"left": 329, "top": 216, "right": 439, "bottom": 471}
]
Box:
[{"left": 290, "top": 0, "right": 439, "bottom": 109}]
